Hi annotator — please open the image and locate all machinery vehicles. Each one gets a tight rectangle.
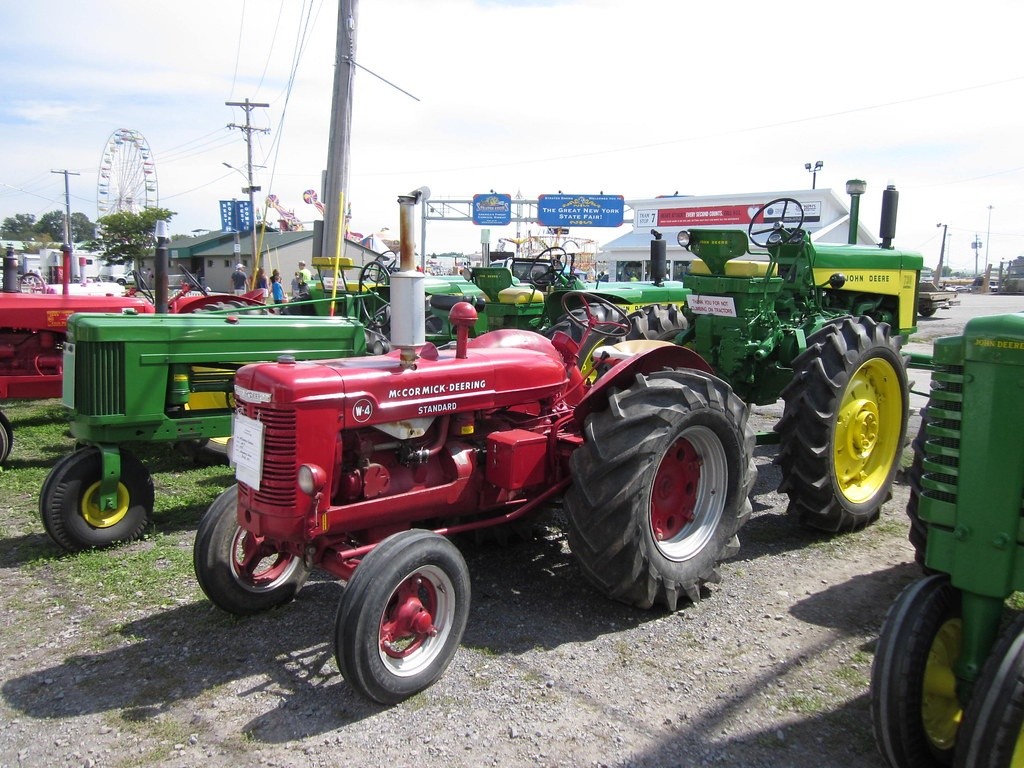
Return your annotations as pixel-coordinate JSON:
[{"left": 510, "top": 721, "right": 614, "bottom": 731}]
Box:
[
  {"left": 917, "top": 222, "right": 960, "bottom": 318},
  {"left": 195, "top": 177, "right": 922, "bottom": 706},
  {"left": 38, "top": 227, "right": 692, "bottom": 554},
  {"left": 867, "top": 314, "right": 1024, "bottom": 768},
  {"left": 0, "top": 244, "right": 279, "bottom": 465}
]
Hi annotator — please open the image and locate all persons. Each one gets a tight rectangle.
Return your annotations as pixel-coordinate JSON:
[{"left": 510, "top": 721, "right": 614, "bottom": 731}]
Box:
[{"left": 227, "top": 260, "right": 311, "bottom": 305}]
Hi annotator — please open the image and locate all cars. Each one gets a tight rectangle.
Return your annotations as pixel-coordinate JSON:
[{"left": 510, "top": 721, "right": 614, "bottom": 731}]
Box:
[
  {"left": 115, "top": 271, "right": 136, "bottom": 285},
  {"left": 939, "top": 278, "right": 997, "bottom": 292}
]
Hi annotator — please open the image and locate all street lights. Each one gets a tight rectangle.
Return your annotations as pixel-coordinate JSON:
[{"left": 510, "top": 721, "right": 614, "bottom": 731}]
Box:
[
  {"left": 224, "top": 162, "right": 258, "bottom": 271},
  {"left": 805, "top": 161, "right": 824, "bottom": 188}
]
